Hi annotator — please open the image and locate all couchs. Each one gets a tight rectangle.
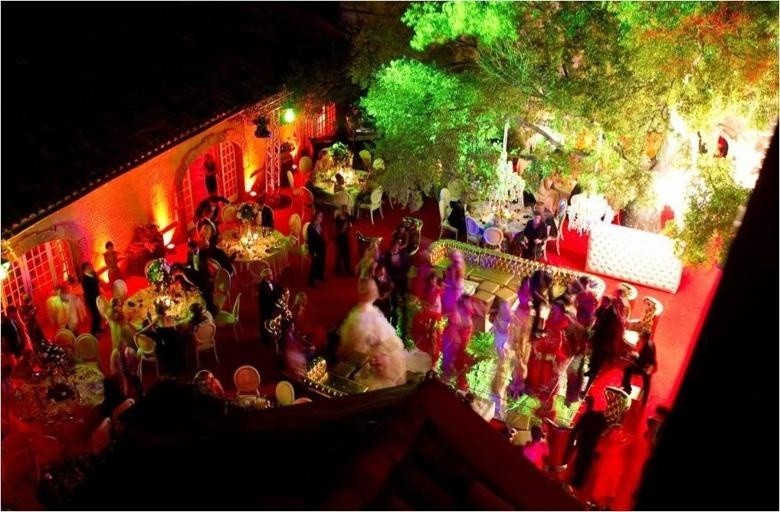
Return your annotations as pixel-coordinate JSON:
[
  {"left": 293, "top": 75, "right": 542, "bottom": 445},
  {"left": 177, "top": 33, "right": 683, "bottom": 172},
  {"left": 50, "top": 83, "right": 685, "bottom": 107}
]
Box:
[{"left": 423, "top": 240, "right": 606, "bottom": 314}]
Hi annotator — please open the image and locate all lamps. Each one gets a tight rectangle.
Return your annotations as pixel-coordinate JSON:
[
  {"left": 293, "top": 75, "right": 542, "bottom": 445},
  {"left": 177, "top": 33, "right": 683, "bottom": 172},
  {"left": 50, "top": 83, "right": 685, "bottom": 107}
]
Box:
[
  {"left": 283, "top": 102, "right": 297, "bottom": 123},
  {"left": 252, "top": 113, "right": 272, "bottom": 137}
]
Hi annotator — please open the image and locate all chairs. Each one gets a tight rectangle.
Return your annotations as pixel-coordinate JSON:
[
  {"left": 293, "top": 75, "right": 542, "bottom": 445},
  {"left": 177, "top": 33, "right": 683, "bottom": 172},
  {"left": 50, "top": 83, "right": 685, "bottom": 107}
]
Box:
[
  {"left": 615, "top": 282, "right": 640, "bottom": 316},
  {"left": 1, "top": 148, "right": 566, "bottom": 510},
  {"left": 594, "top": 385, "right": 632, "bottom": 443},
  {"left": 544, "top": 416, "right": 576, "bottom": 482},
  {"left": 623, "top": 296, "right": 663, "bottom": 362}
]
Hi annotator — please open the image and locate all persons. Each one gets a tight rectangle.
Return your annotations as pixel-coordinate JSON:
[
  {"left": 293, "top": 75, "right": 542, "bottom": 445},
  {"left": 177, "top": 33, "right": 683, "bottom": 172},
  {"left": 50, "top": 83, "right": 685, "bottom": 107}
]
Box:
[
  {"left": 258, "top": 268, "right": 283, "bottom": 346},
  {"left": 519, "top": 212, "right": 547, "bottom": 256},
  {"left": 335, "top": 205, "right": 355, "bottom": 275},
  {"left": 409, "top": 250, "right": 631, "bottom": 377},
  {"left": 382, "top": 241, "right": 411, "bottom": 311},
  {"left": 94, "top": 376, "right": 126, "bottom": 423},
  {"left": 307, "top": 213, "right": 325, "bottom": 286},
  {"left": 253, "top": 200, "right": 274, "bottom": 227},
  {"left": 340, "top": 247, "right": 403, "bottom": 353},
  {"left": 104, "top": 241, "right": 121, "bottom": 284},
  {"left": 569, "top": 396, "right": 606, "bottom": 485},
  {"left": 536, "top": 202, "right": 556, "bottom": 235},
  {"left": 82, "top": 262, "right": 104, "bottom": 338},
  {"left": 140, "top": 236, "right": 242, "bottom": 370},
  {"left": 538, "top": 171, "right": 560, "bottom": 213},
  {"left": 523, "top": 425, "right": 550, "bottom": 469},
  {"left": 448, "top": 199, "right": 466, "bottom": 240},
  {"left": 312, "top": 150, "right": 332, "bottom": 174},
  {"left": 4, "top": 305, "right": 25, "bottom": 357},
  {"left": 622, "top": 330, "right": 654, "bottom": 401}
]
